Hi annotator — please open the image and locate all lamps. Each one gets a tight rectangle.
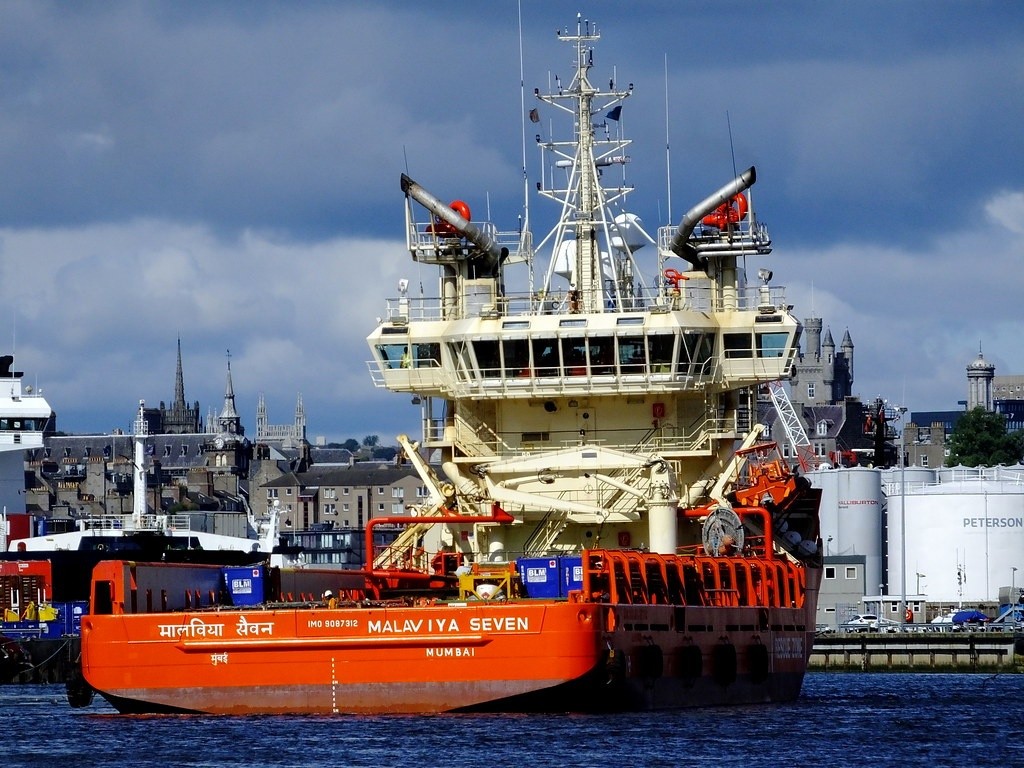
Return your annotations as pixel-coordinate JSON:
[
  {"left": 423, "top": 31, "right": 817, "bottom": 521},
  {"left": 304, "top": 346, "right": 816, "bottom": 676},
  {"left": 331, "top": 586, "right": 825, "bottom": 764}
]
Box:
[
  {"left": 24, "top": 385, "right": 32, "bottom": 395},
  {"left": 479, "top": 310, "right": 500, "bottom": 320},
  {"left": 397, "top": 279, "right": 409, "bottom": 297},
  {"left": 758, "top": 267, "right": 773, "bottom": 285}
]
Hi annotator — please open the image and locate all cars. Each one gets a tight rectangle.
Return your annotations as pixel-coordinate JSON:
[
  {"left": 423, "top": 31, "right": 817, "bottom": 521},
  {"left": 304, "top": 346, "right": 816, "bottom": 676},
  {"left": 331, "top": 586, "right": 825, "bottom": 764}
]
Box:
[{"left": 847, "top": 613, "right": 879, "bottom": 633}]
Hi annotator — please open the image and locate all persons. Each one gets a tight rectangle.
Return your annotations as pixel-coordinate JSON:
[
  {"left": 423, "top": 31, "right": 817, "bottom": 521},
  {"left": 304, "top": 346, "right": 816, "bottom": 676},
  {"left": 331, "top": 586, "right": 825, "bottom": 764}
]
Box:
[
  {"left": 568, "top": 281, "right": 578, "bottom": 309},
  {"left": 399, "top": 347, "right": 414, "bottom": 368}
]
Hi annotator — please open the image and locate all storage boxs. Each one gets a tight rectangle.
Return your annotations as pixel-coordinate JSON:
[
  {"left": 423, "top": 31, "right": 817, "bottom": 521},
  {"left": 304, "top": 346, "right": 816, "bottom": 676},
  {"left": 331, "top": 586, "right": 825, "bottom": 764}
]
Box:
[
  {"left": 220, "top": 565, "right": 281, "bottom": 605},
  {"left": 33, "top": 519, "right": 46, "bottom": 538},
  {"left": 516, "top": 555, "right": 583, "bottom": 599},
  {"left": 3, "top": 600, "right": 86, "bottom": 639}
]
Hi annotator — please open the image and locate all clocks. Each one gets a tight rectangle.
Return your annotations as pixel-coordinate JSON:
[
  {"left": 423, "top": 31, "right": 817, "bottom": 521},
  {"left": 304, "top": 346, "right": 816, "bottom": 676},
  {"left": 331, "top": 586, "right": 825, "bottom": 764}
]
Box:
[{"left": 214, "top": 438, "right": 225, "bottom": 449}]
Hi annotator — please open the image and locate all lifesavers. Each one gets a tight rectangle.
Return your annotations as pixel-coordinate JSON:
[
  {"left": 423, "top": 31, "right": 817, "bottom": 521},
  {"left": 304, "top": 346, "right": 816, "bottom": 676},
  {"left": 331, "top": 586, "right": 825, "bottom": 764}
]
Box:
[
  {"left": 746, "top": 645, "right": 770, "bottom": 684},
  {"left": 64, "top": 660, "right": 93, "bottom": 707},
  {"left": 683, "top": 646, "right": 703, "bottom": 679},
  {"left": 641, "top": 645, "right": 663, "bottom": 680},
  {"left": 711, "top": 644, "right": 738, "bottom": 677},
  {"left": 598, "top": 648, "right": 627, "bottom": 693}
]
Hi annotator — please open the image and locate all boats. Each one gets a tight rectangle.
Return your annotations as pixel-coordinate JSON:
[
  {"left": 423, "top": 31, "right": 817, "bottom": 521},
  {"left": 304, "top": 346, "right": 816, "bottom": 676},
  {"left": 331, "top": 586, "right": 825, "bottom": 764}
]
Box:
[{"left": 0, "top": 0, "right": 826, "bottom": 723}]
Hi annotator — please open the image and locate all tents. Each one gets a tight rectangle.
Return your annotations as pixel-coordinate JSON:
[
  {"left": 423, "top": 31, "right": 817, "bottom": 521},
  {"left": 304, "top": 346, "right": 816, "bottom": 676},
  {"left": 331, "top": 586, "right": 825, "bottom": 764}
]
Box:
[{"left": 953, "top": 611, "right": 990, "bottom": 623}]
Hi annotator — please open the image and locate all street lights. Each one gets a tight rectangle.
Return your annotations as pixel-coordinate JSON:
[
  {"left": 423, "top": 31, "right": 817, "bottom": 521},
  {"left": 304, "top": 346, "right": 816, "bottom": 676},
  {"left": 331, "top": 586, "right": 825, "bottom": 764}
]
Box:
[
  {"left": 878, "top": 584, "right": 884, "bottom": 623},
  {"left": 1012, "top": 567, "right": 1018, "bottom": 632},
  {"left": 916, "top": 572, "right": 926, "bottom": 595}
]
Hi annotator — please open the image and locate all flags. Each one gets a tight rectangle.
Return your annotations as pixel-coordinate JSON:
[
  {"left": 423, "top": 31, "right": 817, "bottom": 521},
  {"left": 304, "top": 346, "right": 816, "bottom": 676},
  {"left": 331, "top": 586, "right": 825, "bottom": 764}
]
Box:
[{"left": 605, "top": 106, "right": 622, "bottom": 121}]
